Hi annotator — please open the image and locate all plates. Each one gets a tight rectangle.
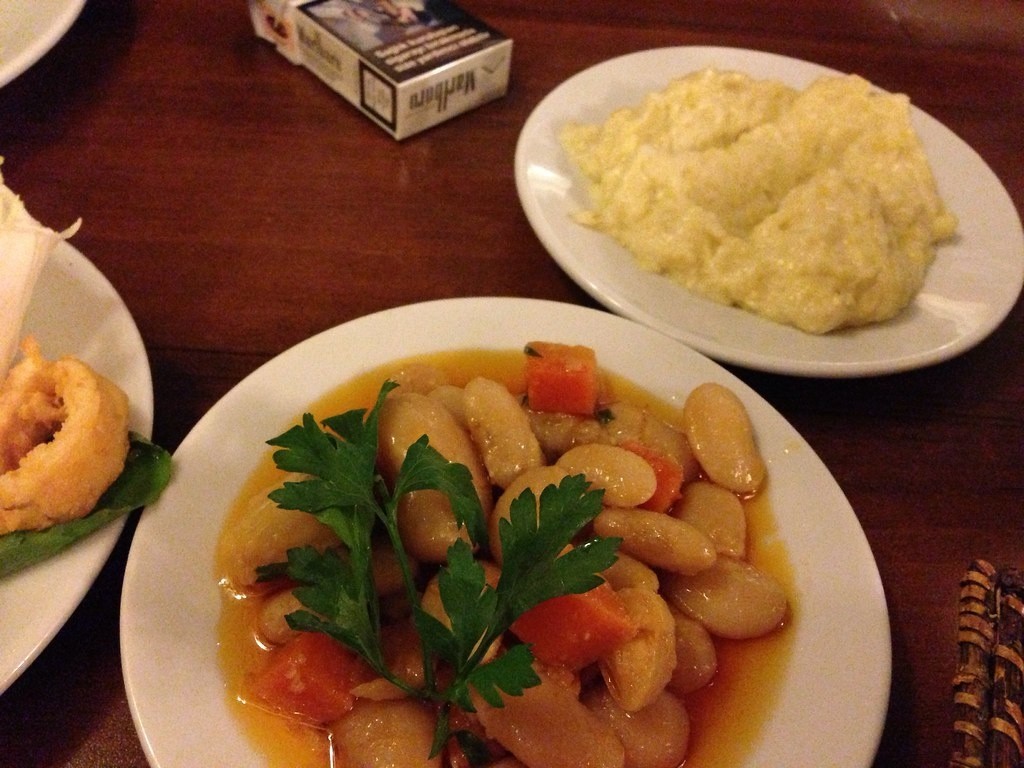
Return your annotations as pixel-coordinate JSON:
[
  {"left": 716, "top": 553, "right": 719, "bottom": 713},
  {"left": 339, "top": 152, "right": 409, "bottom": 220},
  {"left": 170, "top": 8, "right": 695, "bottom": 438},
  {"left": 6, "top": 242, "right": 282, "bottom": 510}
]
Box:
[
  {"left": 515, "top": 44, "right": 1023, "bottom": 387},
  {"left": 119, "top": 297, "right": 894, "bottom": 766},
  {"left": 0, "top": 176, "right": 156, "bottom": 699},
  {"left": 3, "top": 0, "right": 83, "bottom": 91}
]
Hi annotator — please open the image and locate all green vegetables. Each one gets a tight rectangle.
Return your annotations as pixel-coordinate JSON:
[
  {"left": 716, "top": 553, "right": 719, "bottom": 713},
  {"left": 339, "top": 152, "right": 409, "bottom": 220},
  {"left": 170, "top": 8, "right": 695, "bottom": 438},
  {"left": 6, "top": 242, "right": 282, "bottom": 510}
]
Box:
[{"left": 265, "top": 383, "right": 623, "bottom": 768}]
